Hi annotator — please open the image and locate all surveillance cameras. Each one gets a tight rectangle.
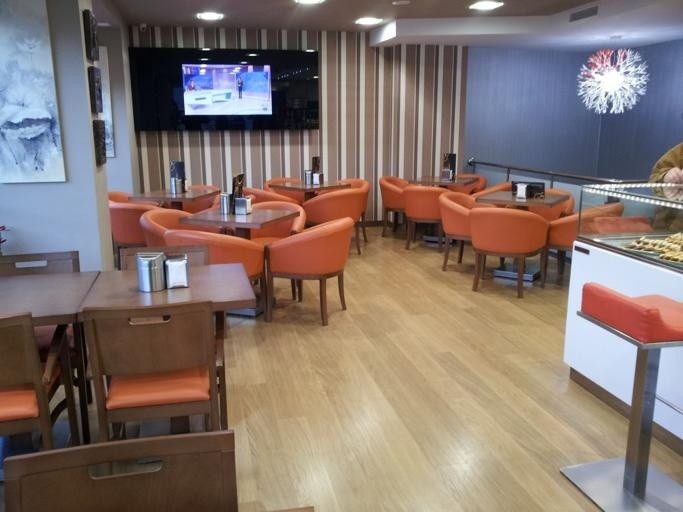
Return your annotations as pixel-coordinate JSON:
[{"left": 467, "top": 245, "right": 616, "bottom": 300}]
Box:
[{"left": 140, "top": 23, "right": 147, "bottom": 32}]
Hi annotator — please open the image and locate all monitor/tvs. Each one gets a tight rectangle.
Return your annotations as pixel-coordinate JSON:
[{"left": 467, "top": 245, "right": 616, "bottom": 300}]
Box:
[{"left": 128, "top": 47, "right": 319, "bottom": 131}]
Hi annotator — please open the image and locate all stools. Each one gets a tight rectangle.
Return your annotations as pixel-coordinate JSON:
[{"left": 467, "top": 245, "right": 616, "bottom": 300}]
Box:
[{"left": 556, "top": 280, "right": 683, "bottom": 512}]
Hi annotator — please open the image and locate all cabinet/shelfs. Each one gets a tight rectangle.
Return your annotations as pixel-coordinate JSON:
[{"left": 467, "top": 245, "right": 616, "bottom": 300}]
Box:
[{"left": 563, "top": 180, "right": 683, "bottom": 462}]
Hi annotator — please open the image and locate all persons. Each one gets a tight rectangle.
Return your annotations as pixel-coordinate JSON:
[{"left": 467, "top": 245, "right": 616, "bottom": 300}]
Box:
[
  {"left": 185, "top": 79, "right": 197, "bottom": 90},
  {"left": 236, "top": 75, "right": 244, "bottom": 97},
  {"left": 649, "top": 144, "right": 683, "bottom": 234}
]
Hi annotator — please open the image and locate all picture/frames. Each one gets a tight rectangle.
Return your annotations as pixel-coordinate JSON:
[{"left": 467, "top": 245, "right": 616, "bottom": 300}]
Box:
[
  {"left": 0, "top": 1, "right": 66, "bottom": 184},
  {"left": 98, "top": 46, "right": 115, "bottom": 159}
]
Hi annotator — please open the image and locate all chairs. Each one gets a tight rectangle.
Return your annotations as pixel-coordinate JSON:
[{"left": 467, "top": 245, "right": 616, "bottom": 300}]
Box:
[
  {"left": 438, "top": 191, "right": 497, "bottom": 272},
  {"left": 106, "top": 192, "right": 157, "bottom": 272},
  {"left": 139, "top": 207, "right": 222, "bottom": 255},
  {"left": 548, "top": 202, "right": 626, "bottom": 279},
  {"left": 117, "top": 243, "right": 210, "bottom": 270},
  {"left": 544, "top": 188, "right": 575, "bottom": 275},
  {"left": 0, "top": 251, "right": 94, "bottom": 406},
  {"left": 3, "top": 430, "right": 239, "bottom": 511},
  {"left": 263, "top": 217, "right": 356, "bottom": 326},
  {"left": 164, "top": 227, "right": 266, "bottom": 340},
  {"left": 250, "top": 201, "right": 306, "bottom": 302},
  {"left": 303, "top": 189, "right": 368, "bottom": 256},
  {"left": 164, "top": 183, "right": 222, "bottom": 214},
  {"left": 338, "top": 178, "right": 370, "bottom": 243},
  {"left": 81, "top": 299, "right": 220, "bottom": 442},
  {"left": 0, "top": 311, "right": 78, "bottom": 451},
  {"left": 471, "top": 182, "right": 513, "bottom": 265},
  {"left": 239, "top": 188, "right": 303, "bottom": 208},
  {"left": 266, "top": 177, "right": 303, "bottom": 203},
  {"left": 380, "top": 176, "right": 411, "bottom": 237},
  {"left": 401, "top": 185, "right": 458, "bottom": 253},
  {"left": 453, "top": 172, "right": 487, "bottom": 197},
  {"left": 468, "top": 207, "right": 551, "bottom": 298}
]
